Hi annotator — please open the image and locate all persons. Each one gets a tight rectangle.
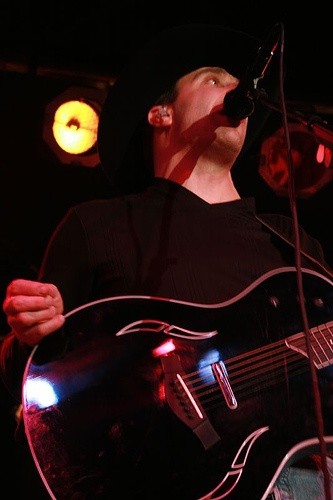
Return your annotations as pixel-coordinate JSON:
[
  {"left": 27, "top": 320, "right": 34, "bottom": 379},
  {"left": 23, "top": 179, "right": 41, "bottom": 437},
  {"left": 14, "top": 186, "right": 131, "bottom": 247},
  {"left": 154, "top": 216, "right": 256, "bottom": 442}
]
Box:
[{"left": 0, "top": 66, "right": 333, "bottom": 500}]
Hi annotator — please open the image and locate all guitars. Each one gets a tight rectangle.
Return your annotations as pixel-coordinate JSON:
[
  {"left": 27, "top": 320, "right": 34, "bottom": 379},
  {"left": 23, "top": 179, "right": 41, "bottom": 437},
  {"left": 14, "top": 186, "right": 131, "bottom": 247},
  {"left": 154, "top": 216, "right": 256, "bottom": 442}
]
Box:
[{"left": 22, "top": 266, "right": 333, "bottom": 500}]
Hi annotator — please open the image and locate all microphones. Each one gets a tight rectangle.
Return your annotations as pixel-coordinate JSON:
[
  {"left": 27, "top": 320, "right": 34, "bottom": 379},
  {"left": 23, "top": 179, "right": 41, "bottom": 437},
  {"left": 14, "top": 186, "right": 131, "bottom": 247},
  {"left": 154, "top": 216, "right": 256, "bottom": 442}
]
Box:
[{"left": 222, "top": 25, "right": 279, "bottom": 118}]
